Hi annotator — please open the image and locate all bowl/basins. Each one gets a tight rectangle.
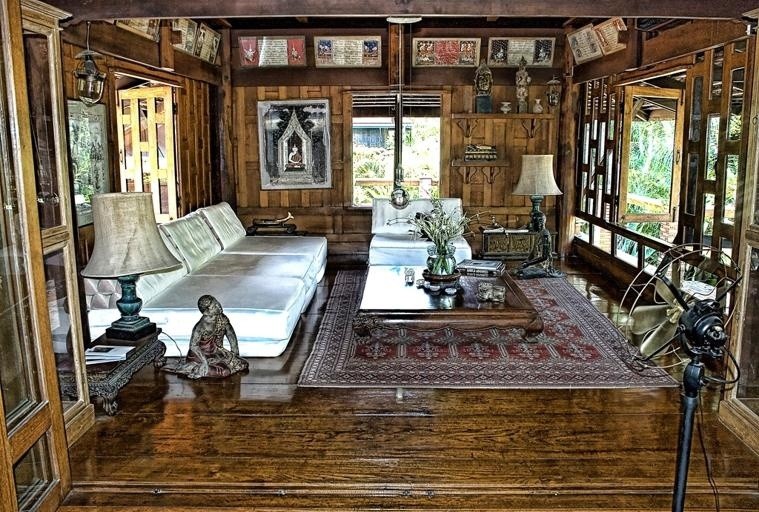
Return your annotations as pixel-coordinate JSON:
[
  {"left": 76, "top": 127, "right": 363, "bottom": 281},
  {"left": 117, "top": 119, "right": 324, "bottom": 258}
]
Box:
[
  {"left": 429, "top": 286, "right": 440, "bottom": 291},
  {"left": 445, "top": 288, "right": 457, "bottom": 295}
]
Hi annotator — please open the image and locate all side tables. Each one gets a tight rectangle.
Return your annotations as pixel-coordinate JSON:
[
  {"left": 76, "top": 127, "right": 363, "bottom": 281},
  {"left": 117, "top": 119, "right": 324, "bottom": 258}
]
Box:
[{"left": 249, "top": 230, "right": 307, "bottom": 235}]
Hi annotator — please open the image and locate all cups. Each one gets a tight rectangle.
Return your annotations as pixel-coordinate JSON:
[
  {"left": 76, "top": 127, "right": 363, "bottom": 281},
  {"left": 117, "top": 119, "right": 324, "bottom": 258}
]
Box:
[
  {"left": 492, "top": 287, "right": 505, "bottom": 304},
  {"left": 478, "top": 283, "right": 493, "bottom": 301},
  {"left": 403, "top": 268, "right": 415, "bottom": 284}
]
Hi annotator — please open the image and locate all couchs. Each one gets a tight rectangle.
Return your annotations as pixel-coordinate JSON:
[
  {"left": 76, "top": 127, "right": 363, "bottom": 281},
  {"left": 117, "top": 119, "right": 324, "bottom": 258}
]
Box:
[
  {"left": 369, "top": 197, "right": 472, "bottom": 265},
  {"left": 83, "top": 201, "right": 327, "bottom": 356}
]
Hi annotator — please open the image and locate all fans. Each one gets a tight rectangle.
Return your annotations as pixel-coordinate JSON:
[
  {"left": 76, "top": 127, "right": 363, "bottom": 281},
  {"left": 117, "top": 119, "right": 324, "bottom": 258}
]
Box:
[{"left": 613, "top": 243, "right": 742, "bottom": 512}]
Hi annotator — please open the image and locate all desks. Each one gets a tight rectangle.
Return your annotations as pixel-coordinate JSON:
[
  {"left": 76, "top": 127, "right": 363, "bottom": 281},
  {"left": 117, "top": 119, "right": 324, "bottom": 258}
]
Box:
[
  {"left": 54, "top": 328, "right": 167, "bottom": 415},
  {"left": 478, "top": 225, "right": 558, "bottom": 265}
]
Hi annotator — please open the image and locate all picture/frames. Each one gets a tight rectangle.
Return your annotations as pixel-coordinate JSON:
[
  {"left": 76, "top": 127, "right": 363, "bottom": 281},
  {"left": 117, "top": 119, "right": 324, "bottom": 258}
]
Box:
[
  {"left": 567, "top": 23, "right": 603, "bottom": 65},
  {"left": 412, "top": 38, "right": 481, "bottom": 66},
  {"left": 117, "top": 18, "right": 162, "bottom": 41},
  {"left": 194, "top": 22, "right": 222, "bottom": 65},
  {"left": 172, "top": 16, "right": 198, "bottom": 54},
  {"left": 238, "top": 35, "right": 307, "bottom": 66},
  {"left": 487, "top": 37, "right": 555, "bottom": 68},
  {"left": 313, "top": 35, "right": 382, "bottom": 68},
  {"left": 257, "top": 99, "right": 331, "bottom": 190},
  {"left": 591, "top": 17, "right": 628, "bottom": 56},
  {"left": 66, "top": 102, "right": 113, "bottom": 230}
]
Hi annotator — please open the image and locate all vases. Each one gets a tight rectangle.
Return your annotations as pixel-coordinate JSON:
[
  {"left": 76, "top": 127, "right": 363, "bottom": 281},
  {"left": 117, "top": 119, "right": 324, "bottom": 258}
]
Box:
[{"left": 423, "top": 246, "right": 460, "bottom": 289}]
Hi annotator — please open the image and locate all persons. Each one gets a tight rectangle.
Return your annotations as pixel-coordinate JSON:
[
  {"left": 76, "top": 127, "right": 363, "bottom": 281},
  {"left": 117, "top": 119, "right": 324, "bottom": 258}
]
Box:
[
  {"left": 508, "top": 211, "right": 567, "bottom": 278},
  {"left": 284, "top": 143, "right": 306, "bottom": 168},
  {"left": 180, "top": 295, "right": 249, "bottom": 379}
]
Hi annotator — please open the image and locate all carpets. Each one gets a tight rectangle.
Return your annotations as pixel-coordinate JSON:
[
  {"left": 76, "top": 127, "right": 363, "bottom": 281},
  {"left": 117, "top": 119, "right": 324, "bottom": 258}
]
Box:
[{"left": 297, "top": 269, "right": 680, "bottom": 387}]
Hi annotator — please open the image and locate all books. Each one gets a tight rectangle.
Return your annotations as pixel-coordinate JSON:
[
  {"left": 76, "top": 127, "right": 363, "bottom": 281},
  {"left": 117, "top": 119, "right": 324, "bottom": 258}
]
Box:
[
  {"left": 457, "top": 259, "right": 502, "bottom": 271},
  {"left": 85, "top": 344, "right": 136, "bottom": 365},
  {"left": 455, "top": 264, "right": 506, "bottom": 277}
]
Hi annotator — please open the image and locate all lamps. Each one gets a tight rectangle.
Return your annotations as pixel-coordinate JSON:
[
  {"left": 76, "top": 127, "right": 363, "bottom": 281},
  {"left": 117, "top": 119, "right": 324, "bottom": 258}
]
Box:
[
  {"left": 81, "top": 194, "right": 182, "bottom": 341},
  {"left": 73, "top": 20, "right": 105, "bottom": 107},
  {"left": 546, "top": 72, "right": 562, "bottom": 107},
  {"left": 510, "top": 154, "right": 564, "bottom": 230}
]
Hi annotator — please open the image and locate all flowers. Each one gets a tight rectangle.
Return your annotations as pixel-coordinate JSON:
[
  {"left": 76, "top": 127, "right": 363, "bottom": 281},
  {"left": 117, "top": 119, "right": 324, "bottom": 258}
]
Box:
[{"left": 386, "top": 188, "right": 510, "bottom": 276}]
